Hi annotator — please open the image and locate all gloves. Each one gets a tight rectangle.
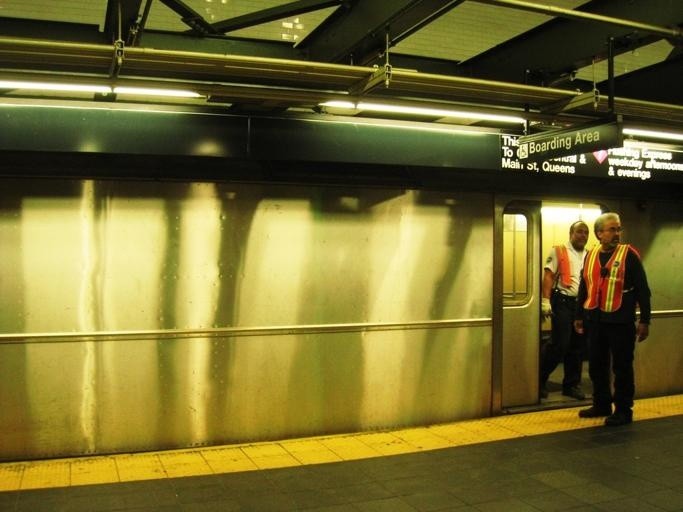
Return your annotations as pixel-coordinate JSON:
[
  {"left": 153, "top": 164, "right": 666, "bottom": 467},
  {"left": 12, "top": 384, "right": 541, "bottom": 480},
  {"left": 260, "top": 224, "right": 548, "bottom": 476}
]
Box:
[{"left": 541, "top": 298, "right": 552, "bottom": 316}]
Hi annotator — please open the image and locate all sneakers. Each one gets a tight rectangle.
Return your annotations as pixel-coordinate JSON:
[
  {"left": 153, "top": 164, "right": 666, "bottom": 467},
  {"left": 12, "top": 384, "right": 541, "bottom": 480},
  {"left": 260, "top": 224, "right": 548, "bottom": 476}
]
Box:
[
  {"left": 562, "top": 385, "right": 584, "bottom": 400},
  {"left": 578, "top": 405, "right": 612, "bottom": 417},
  {"left": 541, "top": 381, "right": 548, "bottom": 397},
  {"left": 605, "top": 409, "right": 632, "bottom": 425}
]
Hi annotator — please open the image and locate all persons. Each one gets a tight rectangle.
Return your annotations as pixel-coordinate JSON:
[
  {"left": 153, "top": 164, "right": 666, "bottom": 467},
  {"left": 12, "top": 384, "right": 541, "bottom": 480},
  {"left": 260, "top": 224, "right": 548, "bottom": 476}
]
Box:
[
  {"left": 540, "top": 220, "right": 588, "bottom": 401},
  {"left": 578, "top": 211, "right": 654, "bottom": 425}
]
